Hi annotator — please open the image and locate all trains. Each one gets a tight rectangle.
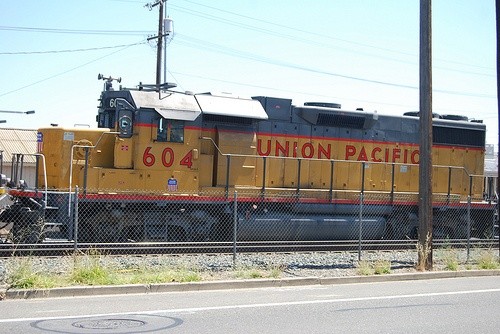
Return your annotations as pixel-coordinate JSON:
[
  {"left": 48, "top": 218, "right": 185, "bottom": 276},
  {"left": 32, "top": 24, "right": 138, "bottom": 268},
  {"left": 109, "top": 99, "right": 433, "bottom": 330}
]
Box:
[{"left": 1, "top": 73, "right": 500, "bottom": 254}]
